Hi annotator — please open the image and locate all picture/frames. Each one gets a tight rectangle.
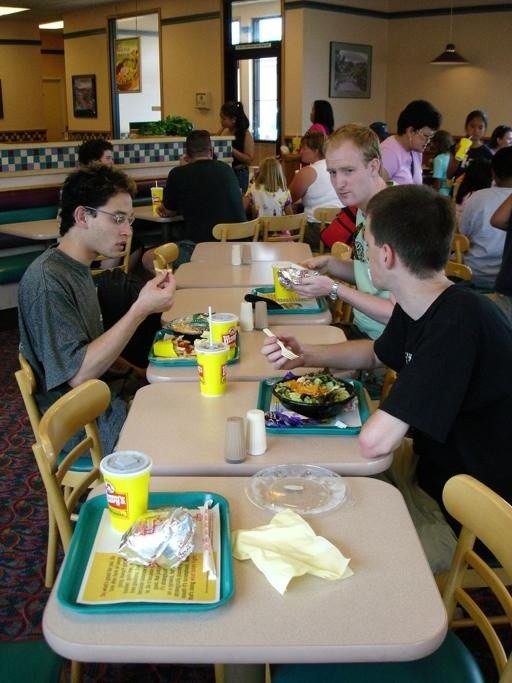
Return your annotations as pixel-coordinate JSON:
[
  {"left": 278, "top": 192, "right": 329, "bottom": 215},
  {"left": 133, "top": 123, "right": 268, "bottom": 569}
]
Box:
[
  {"left": 330, "top": 42, "right": 371, "bottom": 98},
  {"left": 71, "top": 75, "right": 97, "bottom": 118},
  {"left": 114, "top": 37, "right": 141, "bottom": 93}
]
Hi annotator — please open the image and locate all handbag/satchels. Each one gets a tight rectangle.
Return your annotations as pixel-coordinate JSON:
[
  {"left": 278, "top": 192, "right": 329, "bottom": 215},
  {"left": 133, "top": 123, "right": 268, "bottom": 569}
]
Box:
[{"left": 320, "top": 206, "right": 357, "bottom": 250}]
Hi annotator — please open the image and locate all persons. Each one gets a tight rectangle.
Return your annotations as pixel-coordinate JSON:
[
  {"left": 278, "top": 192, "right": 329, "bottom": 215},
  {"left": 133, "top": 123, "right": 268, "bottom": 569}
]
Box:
[
  {"left": 160, "top": 99, "right": 255, "bottom": 268},
  {"left": 56, "top": 138, "right": 122, "bottom": 271},
  {"left": 248, "top": 101, "right": 512, "bottom": 349},
  {"left": 260, "top": 184, "right": 512, "bottom": 621},
  {"left": 14, "top": 163, "right": 176, "bottom": 460}
]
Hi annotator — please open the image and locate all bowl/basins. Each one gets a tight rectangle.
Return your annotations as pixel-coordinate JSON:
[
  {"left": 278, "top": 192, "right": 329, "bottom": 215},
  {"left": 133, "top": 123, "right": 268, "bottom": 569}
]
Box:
[
  {"left": 168, "top": 325, "right": 203, "bottom": 347},
  {"left": 271, "top": 374, "right": 356, "bottom": 420}
]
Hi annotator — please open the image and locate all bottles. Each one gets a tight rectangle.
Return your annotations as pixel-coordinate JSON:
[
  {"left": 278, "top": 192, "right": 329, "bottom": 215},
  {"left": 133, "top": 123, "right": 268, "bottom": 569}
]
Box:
[
  {"left": 254, "top": 300, "right": 269, "bottom": 331},
  {"left": 241, "top": 244, "right": 253, "bottom": 265},
  {"left": 244, "top": 408, "right": 269, "bottom": 456},
  {"left": 231, "top": 245, "right": 241, "bottom": 266},
  {"left": 224, "top": 416, "right": 248, "bottom": 465},
  {"left": 240, "top": 301, "right": 254, "bottom": 332}
]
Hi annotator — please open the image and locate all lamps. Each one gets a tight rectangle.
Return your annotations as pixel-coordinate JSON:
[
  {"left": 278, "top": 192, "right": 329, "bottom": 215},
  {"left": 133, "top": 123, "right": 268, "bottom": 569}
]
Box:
[{"left": 431, "top": 1, "right": 469, "bottom": 64}]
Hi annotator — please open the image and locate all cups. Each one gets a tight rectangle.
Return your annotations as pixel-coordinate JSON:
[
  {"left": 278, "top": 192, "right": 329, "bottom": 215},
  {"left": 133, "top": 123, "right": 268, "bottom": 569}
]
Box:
[
  {"left": 207, "top": 312, "right": 239, "bottom": 362},
  {"left": 292, "top": 137, "right": 301, "bottom": 152},
  {"left": 455, "top": 137, "right": 473, "bottom": 162},
  {"left": 193, "top": 339, "right": 230, "bottom": 398},
  {"left": 150, "top": 187, "right": 164, "bottom": 216},
  {"left": 99, "top": 450, "right": 153, "bottom": 534},
  {"left": 272, "top": 263, "right": 299, "bottom": 302}
]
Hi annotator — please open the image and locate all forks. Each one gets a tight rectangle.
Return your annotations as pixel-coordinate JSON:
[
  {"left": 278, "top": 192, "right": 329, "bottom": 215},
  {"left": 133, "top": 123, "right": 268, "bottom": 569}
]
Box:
[{"left": 262, "top": 328, "right": 299, "bottom": 360}]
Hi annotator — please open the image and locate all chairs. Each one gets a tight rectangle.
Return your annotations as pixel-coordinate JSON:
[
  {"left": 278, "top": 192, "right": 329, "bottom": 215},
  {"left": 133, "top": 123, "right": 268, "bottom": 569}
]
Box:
[
  {"left": 444, "top": 229, "right": 473, "bottom": 288},
  {"left": 271, "top": 474, "right": 511, "bottom": 681}
]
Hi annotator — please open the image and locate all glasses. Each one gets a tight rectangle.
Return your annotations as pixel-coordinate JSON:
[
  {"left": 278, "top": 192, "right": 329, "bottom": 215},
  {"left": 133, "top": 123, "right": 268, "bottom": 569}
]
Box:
[
  {"left": 83, "top": 206, "right": 135, "bottom": 226},
  {"left": 412, "top": 128, "right": 434, "bottom": 139}
]
